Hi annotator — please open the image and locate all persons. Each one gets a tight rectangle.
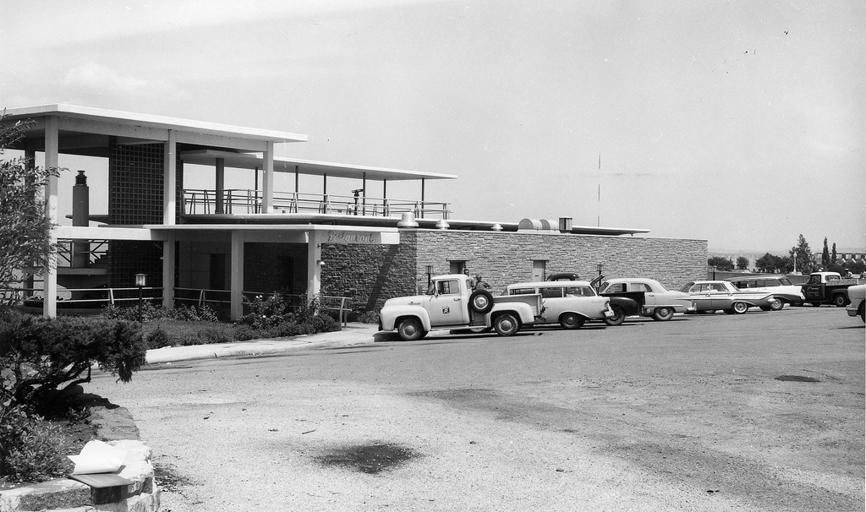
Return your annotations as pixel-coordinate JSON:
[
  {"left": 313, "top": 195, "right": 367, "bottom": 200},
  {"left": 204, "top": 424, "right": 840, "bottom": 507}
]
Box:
[
  {"left": 472, "top": 275, "right": 491, "bottom": 291},
  {"left": 441, "top": 283, "right": 448, "bottom": 293},
  {"left": 844, "top": 269, "right": 853, "bottom": 279}
]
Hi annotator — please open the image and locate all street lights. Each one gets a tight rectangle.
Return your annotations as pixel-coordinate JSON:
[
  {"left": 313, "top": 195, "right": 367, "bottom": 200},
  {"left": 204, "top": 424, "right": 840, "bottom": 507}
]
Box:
[
  {"left": 711, "top": 265, "right": 717, "bottom": 280},
  {"left": 596, "top": 260, "right": 603, "bottom": 287},
  {"left": 425, "top": 264, "right": 433, "bottom": 290},
  {"left": 131, "top": 270, "right": 149, "bottom": 367},
  {"left": 791, "top": 250, "right": 798, "bottom": 275}
]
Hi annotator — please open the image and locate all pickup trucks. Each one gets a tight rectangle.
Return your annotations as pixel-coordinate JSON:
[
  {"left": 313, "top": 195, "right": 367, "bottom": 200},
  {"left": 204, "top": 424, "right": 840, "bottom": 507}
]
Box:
[{"left": 377, "top": 273, "right": 547, "bottom": 345}]
